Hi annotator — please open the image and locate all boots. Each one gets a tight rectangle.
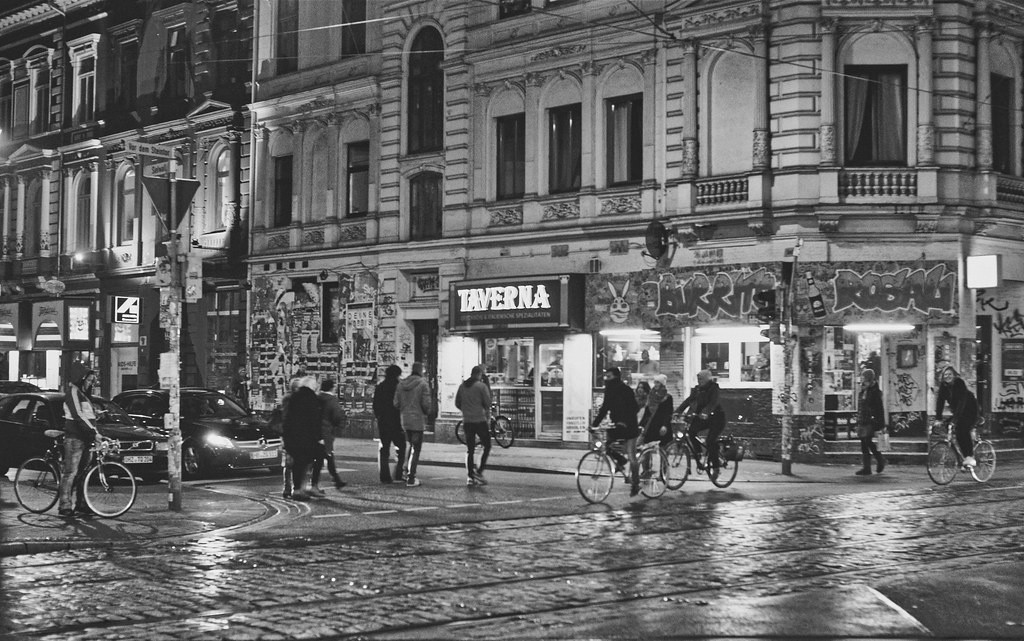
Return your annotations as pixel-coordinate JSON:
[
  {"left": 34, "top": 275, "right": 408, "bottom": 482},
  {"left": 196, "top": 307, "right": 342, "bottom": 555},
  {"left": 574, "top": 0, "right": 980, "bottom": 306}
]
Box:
[{"left": 332, "top": 472, "right": 348, "bottom": 489}]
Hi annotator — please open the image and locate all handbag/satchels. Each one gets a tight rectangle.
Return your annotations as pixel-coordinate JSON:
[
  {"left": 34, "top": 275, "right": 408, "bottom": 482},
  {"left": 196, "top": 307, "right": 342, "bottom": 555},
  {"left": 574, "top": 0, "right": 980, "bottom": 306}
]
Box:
[
  {"left": 878, "top": 432, "right": 891, "bottom": 451},
  {"left": 281, "top": 449, "right": 295, "bottom": 468}
]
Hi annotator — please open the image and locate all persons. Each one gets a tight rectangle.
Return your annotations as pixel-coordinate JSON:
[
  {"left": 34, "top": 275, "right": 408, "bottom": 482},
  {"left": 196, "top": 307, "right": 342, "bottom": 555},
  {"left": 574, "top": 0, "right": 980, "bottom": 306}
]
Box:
[
  {"left": 639, "top": 350, "right": 659, "bottom": 375},
  {"left": 477, "top": 363, "right": 493, "bottom": 449},
  {"left": 632, "top": 381, "right": 652, "bottom": 438},
  {"left": 393, "top": 361, "right": 433, "bottom": 488},
  {"left": 673, "top": 369, "right": 727, "bottom": 474},
  {"left": 588, "top": 367, "right": 642, "bottom": 497},
  {"left": 372, "top": 363, "right": 407, "bottom": 484},
  {"left": 634, "top": 373, "right": 673, "bottom": 483},
  {"left": 854, "top": 368, "right": 886, "bottom": 475},
  {"left": 933, "top": 365, "right": 981, "bottom": 474},
  {"left": 454, "top": 366, "right": 493, "bottom": 486},
  {"left": 280, "top": 374, "right": 348, "bottom": 502},
  {"left": 859, "top": 350, "right": 882, "bottom": 387},
  {"left": 547, "top": 350, "right": 564, "bottom": 425},
  {"left": 57, "top": 362, "right": 104, "bottom": 517}
]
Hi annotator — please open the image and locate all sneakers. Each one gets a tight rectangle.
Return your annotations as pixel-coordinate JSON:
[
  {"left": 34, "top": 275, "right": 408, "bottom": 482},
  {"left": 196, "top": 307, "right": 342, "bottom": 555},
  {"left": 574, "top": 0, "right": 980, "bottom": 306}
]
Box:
[{"left": 961, "top": 456, "right": 976, "bottom": 472}]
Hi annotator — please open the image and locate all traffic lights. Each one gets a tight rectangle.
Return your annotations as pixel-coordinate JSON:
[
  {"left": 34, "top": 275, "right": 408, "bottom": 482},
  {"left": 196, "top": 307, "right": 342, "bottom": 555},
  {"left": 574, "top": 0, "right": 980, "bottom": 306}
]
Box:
[{"left": 753, "top": 290, "right": 775, "bottom": 323}]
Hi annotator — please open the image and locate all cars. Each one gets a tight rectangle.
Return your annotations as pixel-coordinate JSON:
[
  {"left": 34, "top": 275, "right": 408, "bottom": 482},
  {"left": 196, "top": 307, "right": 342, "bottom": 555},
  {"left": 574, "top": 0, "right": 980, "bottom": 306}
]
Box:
[
  {"left": 0, "top": 381, "right": 44, "bottom": 392},
  {"left": 111, "top": 387, "right": 286, "bottom": 480},
  {"left": 1, "top": 392, "right": 170, "bottom": 485}
]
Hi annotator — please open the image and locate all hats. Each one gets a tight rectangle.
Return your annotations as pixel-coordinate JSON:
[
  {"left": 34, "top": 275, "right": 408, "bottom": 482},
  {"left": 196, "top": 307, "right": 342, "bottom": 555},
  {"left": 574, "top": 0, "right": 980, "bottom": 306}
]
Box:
[
  {"left": 298, "top": 376, "right": 316, "bottom": 391},
  {"left": 699, "top": 370, "right": 712, "bottom": 383},
  {"left": 653, "top": 374, "right": 667, "bottom": 386},
  {"left": 289, "top": 378, "right": 301, "bottom": 392},
  {"left": 320, "top": 380, "right": 337, "bottom": 391},
  {"left": 862, "top": 369, "right": 875, "bottom": 382},
  {"left": 412, "top": 362, "right": 423, "bottom": 376}
]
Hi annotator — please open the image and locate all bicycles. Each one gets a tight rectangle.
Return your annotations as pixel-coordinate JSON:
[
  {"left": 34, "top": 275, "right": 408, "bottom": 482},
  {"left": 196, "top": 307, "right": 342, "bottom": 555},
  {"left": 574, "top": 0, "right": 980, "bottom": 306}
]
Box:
[
  {"left": 14, "top": 429, "right": 138, "bottom": 519},
  {"left": 659, "top": 413, "right": 746, "bottom": 491},
  {"left": 455, "top": 402, "right": 515, "bottom": 448},
  {"left": 926, "top": 414, "right": 996, "bottom": 486},
  {"left": 575, "top": 422, "right": 670, "bottom": 504}
]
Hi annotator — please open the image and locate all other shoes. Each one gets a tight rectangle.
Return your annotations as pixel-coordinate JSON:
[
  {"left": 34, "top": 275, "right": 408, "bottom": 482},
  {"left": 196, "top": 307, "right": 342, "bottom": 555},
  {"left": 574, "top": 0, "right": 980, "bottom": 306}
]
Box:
[
  {"left": 856, "top": 466, "right": 872, "bottom": 475},
  {"left": 282, "top": 485, "right": 292, "bottom": 498},
  {"left": 293, "top": 488, "right": 311, "bottom": 499},
  {"left": 74, "top": 506, "right": 96, "bottom": 515},
  {"left": 657, "top": 475, "right": 663, "bottom": 481},
  {"left": 406, "top": 477, "right": 423, "bottom": 486},
  {"left": 471, "top": 471, "right": 488, "bottom": 484},
  {"left": 58, "top": 509, "right": 79, "bottom": 517},
  {"left": 639, "top": 469, "right": 651, "bottom": 478},
  {"left": 630, "top": 480, "right": 640, "bottom": 495},
  {"left": 393, "top": 477, "right": 407, "bottom": 483},
  {"left": 615, "top": 455, "right": 627, "bottom": 473},
  {"left": 466, "top": 477, "right": 478, "bottom": 486},
  {"left": 310, "top": 486, "right": 325, "bottom": 496},
  {"left": 712, "top": 468, "right": 719, "bottom": 479},
  {"left": 877, "top": 457, "right": 889, "bottom": 473}
]
